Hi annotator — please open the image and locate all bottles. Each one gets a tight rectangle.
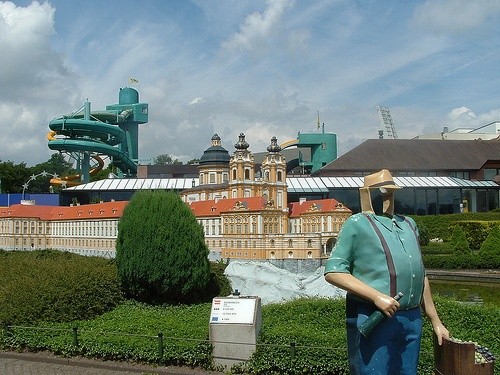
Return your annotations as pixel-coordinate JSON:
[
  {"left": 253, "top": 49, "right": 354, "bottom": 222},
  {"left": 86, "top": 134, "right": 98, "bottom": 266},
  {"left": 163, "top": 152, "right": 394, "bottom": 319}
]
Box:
[{"left": 357, "top": 292, "right": 404, "bottom": 337}]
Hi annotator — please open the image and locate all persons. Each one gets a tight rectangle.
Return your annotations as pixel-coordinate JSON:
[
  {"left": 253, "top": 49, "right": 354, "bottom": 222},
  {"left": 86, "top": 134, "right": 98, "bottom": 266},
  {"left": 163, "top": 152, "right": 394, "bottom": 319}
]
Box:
[{"left": 323, "top": 168, "right": 450, "bottom": 375}]
